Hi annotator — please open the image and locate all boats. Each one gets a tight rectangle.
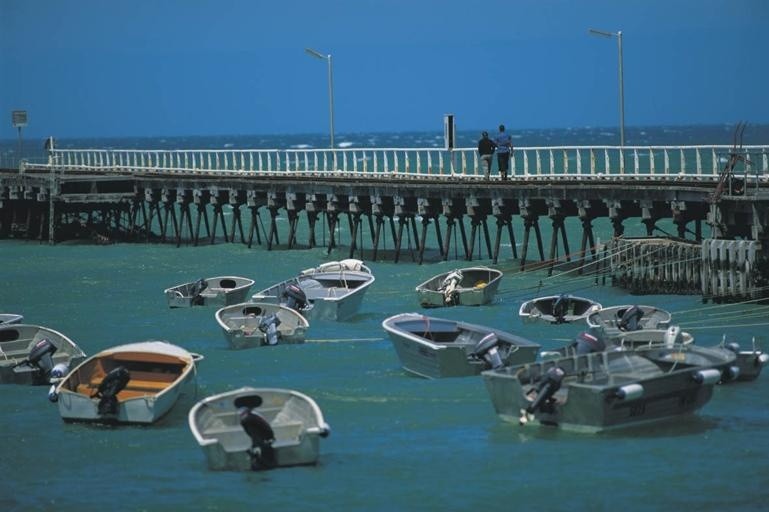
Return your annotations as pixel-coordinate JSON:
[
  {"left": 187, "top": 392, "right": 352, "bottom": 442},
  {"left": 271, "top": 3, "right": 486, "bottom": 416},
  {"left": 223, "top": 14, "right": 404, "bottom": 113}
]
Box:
[
  {"left": 60, "top": 335, "right": 194, "bottom": 424},
  {"left": 163, "top": 274, "right": 252, "bottom": 306},
  {"left": 385, "top": 311, "right": 538, "bottom": 374},
  {"left": 188, "top": 386, "right": 327, "bottom": 468},
  {"left": 254, "top": 257, "right": 381, "bottom": 318},
  {"left": 523, "top": 294, "right": 685, "bottom": 348},
  {"left": 213, "top": 301, "right": 307, "bottom": 345},
  {"left": 1, "top": 324, "right": 81, "bottom": 387},
  {"left": 407, "top": 262, "right": 502, "bottom": 305},
  {"left": 482, "top": 348, "right": 735, "bottom": 427}
]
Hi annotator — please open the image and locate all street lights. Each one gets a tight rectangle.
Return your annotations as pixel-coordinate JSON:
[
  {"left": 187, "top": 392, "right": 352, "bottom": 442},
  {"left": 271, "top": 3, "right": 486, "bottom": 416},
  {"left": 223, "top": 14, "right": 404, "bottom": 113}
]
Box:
[
  {"left": 587, "top": 26, "right": 627, "bottom": 148},
  {"left": 308, "top": 42, "right": 337, "bottom": 152}
]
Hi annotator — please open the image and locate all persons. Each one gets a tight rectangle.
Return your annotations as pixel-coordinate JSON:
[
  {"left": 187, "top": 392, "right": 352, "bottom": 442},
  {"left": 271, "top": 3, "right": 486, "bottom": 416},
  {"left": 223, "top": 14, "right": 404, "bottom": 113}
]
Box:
[
  {"left": 493, "top": 125, "right": 514, "bottom": 180},
  {"left": 478, "top": 131, "right": 498, "bottom": 180}
]
